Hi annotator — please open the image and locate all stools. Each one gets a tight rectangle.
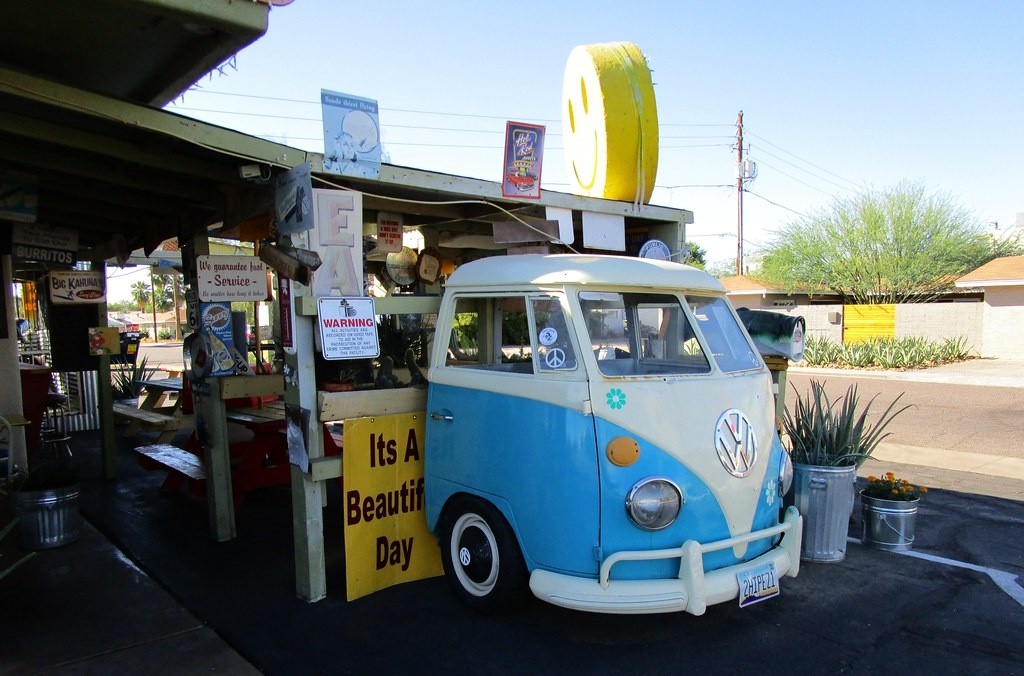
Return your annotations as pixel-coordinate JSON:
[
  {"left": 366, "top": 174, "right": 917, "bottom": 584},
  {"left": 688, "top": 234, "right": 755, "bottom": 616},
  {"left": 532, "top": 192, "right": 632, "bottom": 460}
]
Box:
[{"left": 44, "top": 392, "right": 75, "bottom": 457}]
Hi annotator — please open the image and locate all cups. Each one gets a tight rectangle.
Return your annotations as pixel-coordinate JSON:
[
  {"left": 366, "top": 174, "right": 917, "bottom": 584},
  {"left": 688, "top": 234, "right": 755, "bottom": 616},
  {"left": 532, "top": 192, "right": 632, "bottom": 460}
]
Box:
[{"left": 72, "top": 285, "right": 105, "bottom": 302}]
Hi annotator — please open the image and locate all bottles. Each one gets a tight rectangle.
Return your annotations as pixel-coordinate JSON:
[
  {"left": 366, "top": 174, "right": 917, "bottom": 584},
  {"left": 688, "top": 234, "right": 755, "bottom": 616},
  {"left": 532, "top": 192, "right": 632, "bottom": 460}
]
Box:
[{"left": 204, "top": 323, "right": 234, "bottom": 371}]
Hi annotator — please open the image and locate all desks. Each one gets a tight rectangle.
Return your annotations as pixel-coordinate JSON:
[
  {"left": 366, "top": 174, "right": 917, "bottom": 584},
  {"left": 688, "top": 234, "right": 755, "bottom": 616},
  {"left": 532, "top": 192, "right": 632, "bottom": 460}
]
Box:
[
  {"left": 158, "top": 401, "right": 291, "bottom": 512},
  {"left": 123, "top": 376, "right": 187, "bottom": 448}
]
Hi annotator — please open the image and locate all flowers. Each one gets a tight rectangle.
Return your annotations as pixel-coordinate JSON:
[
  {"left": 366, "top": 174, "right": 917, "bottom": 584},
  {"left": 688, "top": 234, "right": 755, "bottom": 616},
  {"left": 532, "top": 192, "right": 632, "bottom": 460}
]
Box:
[{"left": 865, "top": 471, "right": 928, "bottom": 499}]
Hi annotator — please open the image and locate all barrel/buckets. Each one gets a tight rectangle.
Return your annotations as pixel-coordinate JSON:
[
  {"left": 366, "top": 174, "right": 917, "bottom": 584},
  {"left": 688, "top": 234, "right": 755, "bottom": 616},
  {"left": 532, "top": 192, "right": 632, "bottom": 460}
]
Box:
[{"left": 14, "top": 481, "right": 81, "bottom": 551}]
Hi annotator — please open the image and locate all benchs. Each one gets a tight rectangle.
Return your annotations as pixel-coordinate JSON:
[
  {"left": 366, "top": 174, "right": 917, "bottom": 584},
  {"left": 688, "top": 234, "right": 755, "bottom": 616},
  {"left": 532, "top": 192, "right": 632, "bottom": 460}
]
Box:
[{"left": 104, "top": 403, "right": 358, "bottom": 488}]
